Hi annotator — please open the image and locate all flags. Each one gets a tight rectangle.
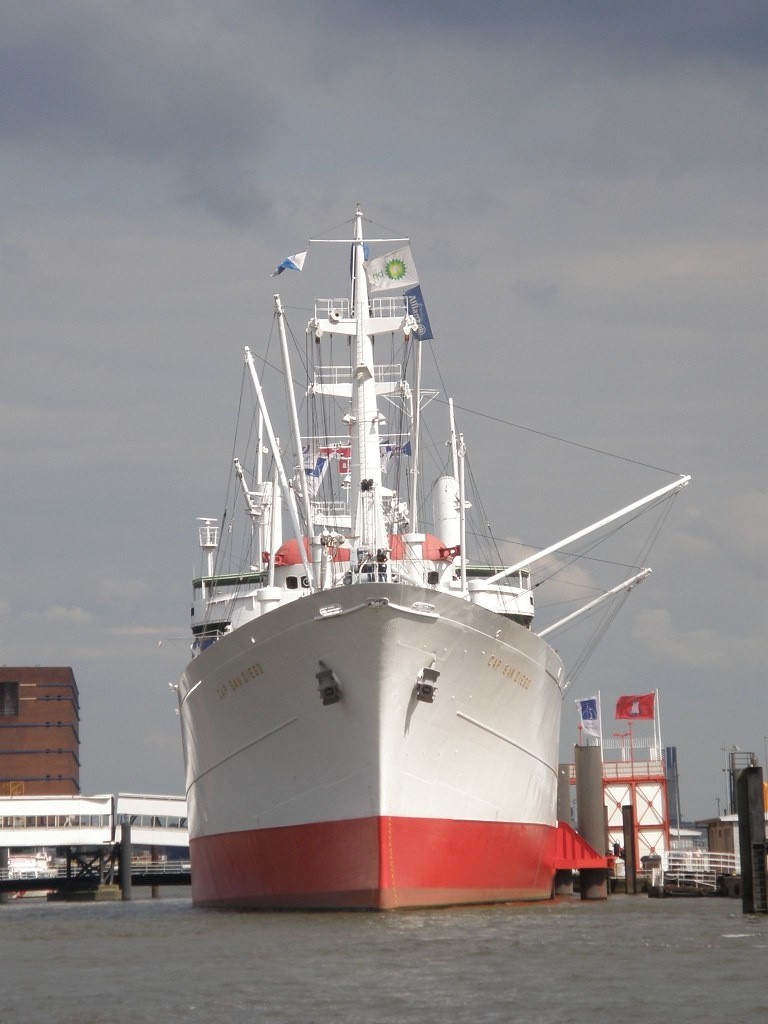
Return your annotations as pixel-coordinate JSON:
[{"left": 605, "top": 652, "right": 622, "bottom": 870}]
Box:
[
  {"left": 615, "top": 692, "right": 657, "bottom": 720},
  {"left": 363, "top": 244, "right": 418, "bottom": 292},
  {"left": 270, "top": 252, "right": 306, "bottom": 276}
]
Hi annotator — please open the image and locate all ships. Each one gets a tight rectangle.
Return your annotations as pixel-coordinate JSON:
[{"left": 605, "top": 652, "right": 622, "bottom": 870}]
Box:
[{"left": 166, "top": 202, "right": 692, "bottom": 915}]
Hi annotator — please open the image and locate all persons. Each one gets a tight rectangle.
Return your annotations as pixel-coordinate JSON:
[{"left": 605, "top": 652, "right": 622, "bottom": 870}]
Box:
[{"left": 377, "top": 549, "right": 388, "bottom": 572}]
